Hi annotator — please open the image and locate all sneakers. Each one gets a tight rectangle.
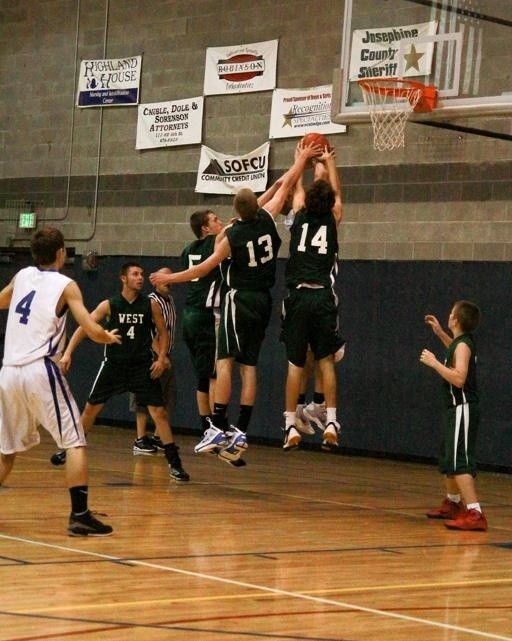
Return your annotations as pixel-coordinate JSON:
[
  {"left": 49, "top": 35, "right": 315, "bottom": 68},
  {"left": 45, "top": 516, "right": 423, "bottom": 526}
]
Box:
[
  {"left": 283, "top": 402, "right": 342, "bottom": 434},
  {"left": 283, "top": 426, "right": 302, "bottom": 452},
  {"left": 427, "top": 497, "right": 463, "bottom": 518},
  {"left": 50, "top": 448, "right": 68, "bottom": 465},
  {"left": 168, "top": 461, "right": 189, "bottom": 482},
  {"left": 68, "top": 511, "right": 113, "bottom": 536},
  {"left": 133, "top": 435, "right": 179, "bottom": 453},
  {"left": 443, "top": 507, "right": 488, "bottom": 531},
  {"left": 195, "top": 417, "right": 248, "bottom": 468},
  {"left": 322, "top": 421, "right": 338, "bottom": 451}
]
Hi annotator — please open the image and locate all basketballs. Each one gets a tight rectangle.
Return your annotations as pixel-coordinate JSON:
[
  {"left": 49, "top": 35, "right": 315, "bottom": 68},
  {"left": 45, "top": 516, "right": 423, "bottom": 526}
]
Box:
[{"left": 303, "top": 133, "right": 329, "bottom": 153}]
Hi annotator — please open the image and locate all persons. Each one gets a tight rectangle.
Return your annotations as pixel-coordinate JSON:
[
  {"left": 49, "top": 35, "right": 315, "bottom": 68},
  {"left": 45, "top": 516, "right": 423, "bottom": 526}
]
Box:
[
  {"left": 52, "top": 265, "right": 190, "bottom": 484},
  {"left": 130, "top": 270, "right": 185, "bottom": 455},
  {"left": 417, "top": 300, "right": 494, "bottom": 534},
  {"left": 0, "top": 226, "right": 117, "bottom": 534}
]
